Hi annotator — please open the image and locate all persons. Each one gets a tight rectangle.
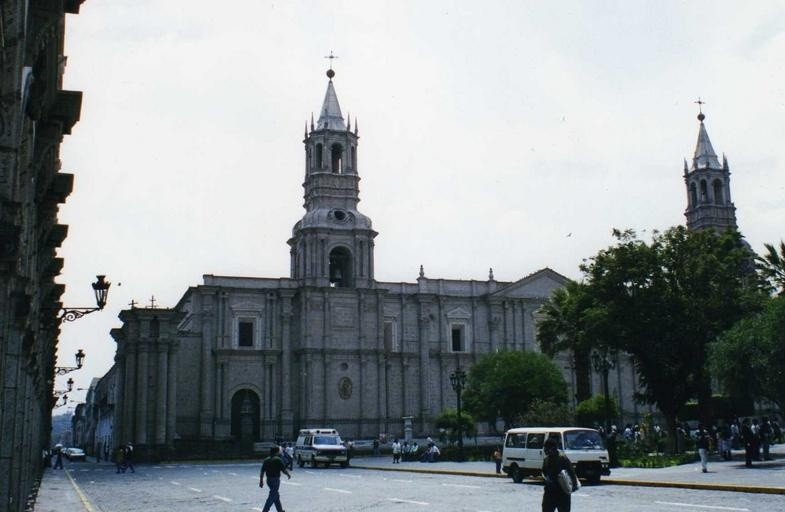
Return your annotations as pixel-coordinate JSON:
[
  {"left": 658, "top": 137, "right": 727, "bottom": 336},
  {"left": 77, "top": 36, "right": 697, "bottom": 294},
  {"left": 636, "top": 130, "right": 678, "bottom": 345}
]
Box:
[
  {"left": 278, "top": 441, "right": 294, "bottom": 471},
  {"left": 592, "top": 415, "right": 785, "bottom": 474},
  {"left": 373, "top": 431, "right": 440, "bottom": 463},
  {"left": 259, "top": 446, "right": 291, "bottom": 512},
  {"left": 539, "top": 438, "right": 577, "bottom": 512},
  {"left": 343, "top": 438, "right": 352, "bottom": 465},
  {"left": 116, "top": 441, "right": 136, "bottom": 474},
  {"left": 494, "top": 446, "right": 502, "bottom": 474},
  {"left": 53, "top": 448, "right": 64, "bottom": 470}
]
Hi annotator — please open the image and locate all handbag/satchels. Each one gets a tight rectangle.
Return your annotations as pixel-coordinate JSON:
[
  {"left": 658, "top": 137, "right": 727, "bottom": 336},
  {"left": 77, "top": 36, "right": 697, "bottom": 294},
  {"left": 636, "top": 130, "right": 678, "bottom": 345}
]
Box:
[{"left": 559, "top": 469, "right": 582, "bottom": 495}]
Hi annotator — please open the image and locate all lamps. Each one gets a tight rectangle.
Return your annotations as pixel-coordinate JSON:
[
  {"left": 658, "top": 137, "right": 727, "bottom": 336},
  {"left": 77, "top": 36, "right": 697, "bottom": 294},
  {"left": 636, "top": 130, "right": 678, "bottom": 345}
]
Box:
[
  {"left": 39, "top": 272, "right": 112, "bottom": 332},
  {"left": 49, "top": 350, "right": 85, "bottom": 409}
]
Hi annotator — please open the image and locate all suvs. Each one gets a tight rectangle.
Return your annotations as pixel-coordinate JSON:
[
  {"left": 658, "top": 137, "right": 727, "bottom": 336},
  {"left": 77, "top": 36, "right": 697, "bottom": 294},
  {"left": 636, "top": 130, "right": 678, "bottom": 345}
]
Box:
[{"left": 295, "top": 429, "right": 348, "bottom": 468}]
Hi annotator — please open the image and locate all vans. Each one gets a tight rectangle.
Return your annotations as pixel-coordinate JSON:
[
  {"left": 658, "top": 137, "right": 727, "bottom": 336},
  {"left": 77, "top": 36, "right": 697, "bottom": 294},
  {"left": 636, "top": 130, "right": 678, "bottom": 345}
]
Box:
[{"left": 502, "top": 426, "right": 611, "bottom": 483}]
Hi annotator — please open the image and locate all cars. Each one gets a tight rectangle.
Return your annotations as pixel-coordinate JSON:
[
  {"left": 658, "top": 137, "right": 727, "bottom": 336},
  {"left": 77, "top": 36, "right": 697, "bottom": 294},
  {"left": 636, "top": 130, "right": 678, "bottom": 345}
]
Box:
[{"left": 61, "top": 448, "right": 85, "bottom": 459}]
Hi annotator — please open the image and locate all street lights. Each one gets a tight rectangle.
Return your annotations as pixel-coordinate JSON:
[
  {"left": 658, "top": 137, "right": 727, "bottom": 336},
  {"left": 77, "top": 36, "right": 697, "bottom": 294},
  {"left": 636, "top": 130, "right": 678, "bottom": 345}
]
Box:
[
  {"left": 68, "top": 387, "right": 94, "bottom": 419},
  {"left": 449, "top": 367, "right": 466, "bottom": 449}
]
